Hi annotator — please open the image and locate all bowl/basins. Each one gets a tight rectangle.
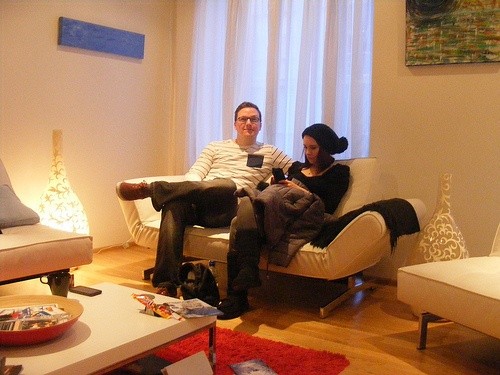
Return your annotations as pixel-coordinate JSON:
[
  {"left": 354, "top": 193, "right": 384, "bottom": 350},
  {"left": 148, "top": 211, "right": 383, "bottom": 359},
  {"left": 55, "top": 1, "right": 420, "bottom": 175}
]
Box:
[{"left": 0, "top": 294, "right": 84, "bottom": 345}]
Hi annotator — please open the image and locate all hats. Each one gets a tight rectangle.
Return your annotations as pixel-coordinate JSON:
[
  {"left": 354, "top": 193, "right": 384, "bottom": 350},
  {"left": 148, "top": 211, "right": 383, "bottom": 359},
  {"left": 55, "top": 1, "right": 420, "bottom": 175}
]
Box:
[{"left": 303, "top": 123, "right": 348, "bottom": 154}]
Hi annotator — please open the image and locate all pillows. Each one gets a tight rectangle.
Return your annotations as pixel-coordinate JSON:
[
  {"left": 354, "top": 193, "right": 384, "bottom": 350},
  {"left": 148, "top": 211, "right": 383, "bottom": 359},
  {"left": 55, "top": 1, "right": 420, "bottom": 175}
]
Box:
[{"left": 0, "top": 158, "right": 41, "bottom": 229}]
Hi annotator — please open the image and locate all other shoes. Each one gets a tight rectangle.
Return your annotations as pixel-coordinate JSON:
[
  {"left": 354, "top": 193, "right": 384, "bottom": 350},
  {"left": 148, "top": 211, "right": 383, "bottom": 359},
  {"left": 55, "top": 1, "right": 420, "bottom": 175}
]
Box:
[
  {"left": 237, "top": 268, "right": 260, "bottom": 286},
  {"left": 155, "top": 284, "right": 177, "bottom": 296},
  {"left": 217, "top": 298, "right": 247, "bottom": 320},
  {"left": 116, "top": 180, "right": 149, "bottom": 201}
]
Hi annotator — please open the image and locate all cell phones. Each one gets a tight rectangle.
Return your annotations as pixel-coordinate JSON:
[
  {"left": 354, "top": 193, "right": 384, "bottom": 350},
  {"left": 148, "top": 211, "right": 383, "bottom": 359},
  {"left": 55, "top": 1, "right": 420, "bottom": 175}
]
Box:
[
  {"left": 273, "top": 168, "right": 287, "bottom": 184},
  {"left": 69, "top": 286, "right": 102, "bottom": 297}
]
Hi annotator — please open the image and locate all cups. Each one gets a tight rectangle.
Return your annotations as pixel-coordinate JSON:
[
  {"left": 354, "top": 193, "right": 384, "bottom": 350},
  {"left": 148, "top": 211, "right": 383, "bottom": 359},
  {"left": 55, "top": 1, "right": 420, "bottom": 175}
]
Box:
[{"left": 40, "top": 271, "right": 71, "bottom": 297}]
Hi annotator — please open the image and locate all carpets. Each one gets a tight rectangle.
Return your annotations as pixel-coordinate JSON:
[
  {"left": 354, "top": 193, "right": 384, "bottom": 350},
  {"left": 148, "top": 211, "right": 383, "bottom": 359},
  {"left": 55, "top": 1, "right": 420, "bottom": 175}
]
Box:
[{"left": 155, "top": 325, "right": 350, "bottom": 375}]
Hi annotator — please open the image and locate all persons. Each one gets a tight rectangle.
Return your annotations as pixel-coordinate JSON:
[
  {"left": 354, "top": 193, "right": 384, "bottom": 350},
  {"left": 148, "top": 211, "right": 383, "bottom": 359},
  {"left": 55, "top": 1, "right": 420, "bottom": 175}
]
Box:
[
  {"left": 217, "top": 124, "right": 351, "bottom": 319},
  {"left": 114, "top": 102, "right": 296, "bottom": 298}
]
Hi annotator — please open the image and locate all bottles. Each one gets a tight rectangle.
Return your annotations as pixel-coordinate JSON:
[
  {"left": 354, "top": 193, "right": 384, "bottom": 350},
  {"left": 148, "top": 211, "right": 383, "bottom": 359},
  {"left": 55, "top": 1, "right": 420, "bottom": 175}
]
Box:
[{"left": 208, "top": 260, "right": 219, "bottom": 287}]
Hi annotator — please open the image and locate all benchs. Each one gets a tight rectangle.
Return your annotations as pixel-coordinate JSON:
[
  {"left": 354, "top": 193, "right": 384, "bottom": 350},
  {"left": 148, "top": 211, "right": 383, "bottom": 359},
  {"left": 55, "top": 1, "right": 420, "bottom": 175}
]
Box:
[
  {"left": 395, "top": 256, "right": 500, "bottom": 351},
  {"left": 116, "top": 155, "right": 428, "bottom": 320}
]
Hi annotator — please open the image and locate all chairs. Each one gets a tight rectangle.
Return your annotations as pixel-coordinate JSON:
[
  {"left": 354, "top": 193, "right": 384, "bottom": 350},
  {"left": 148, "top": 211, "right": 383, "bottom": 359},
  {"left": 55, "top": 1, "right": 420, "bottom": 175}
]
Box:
[{"left": 0, "top": 223, "right": 94, "bottom": 296}]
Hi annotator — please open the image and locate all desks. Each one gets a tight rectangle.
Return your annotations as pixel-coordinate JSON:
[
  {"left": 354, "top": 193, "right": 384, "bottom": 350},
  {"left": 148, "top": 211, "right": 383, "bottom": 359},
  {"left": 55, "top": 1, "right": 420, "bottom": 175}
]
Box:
[{"left": 0, "top": 283, "right": 217, "bottom": 375}]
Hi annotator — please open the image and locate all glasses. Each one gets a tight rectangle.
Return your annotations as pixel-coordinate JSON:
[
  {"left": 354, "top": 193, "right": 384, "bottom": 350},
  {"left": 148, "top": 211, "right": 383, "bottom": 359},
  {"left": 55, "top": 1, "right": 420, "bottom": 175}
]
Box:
[{"left": 236, "top": 116, "right": 261, "bottom": 124}]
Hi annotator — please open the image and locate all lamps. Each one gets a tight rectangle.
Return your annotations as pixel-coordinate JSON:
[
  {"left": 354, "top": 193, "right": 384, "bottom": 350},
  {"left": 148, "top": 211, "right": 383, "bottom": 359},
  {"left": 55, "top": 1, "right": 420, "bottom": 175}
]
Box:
[
  {"left": 39, "top": 129, "right": 91, "bottom": 236},
  {"left": 405, "top": 172, "right": 470, "bottom": 323}
]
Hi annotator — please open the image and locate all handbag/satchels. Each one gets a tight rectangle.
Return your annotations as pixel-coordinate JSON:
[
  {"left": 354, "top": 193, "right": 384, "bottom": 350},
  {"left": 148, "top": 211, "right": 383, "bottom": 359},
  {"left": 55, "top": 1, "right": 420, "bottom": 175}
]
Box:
[{"left": 179, "top": 261, "right": 220, "bottom": 305}]
